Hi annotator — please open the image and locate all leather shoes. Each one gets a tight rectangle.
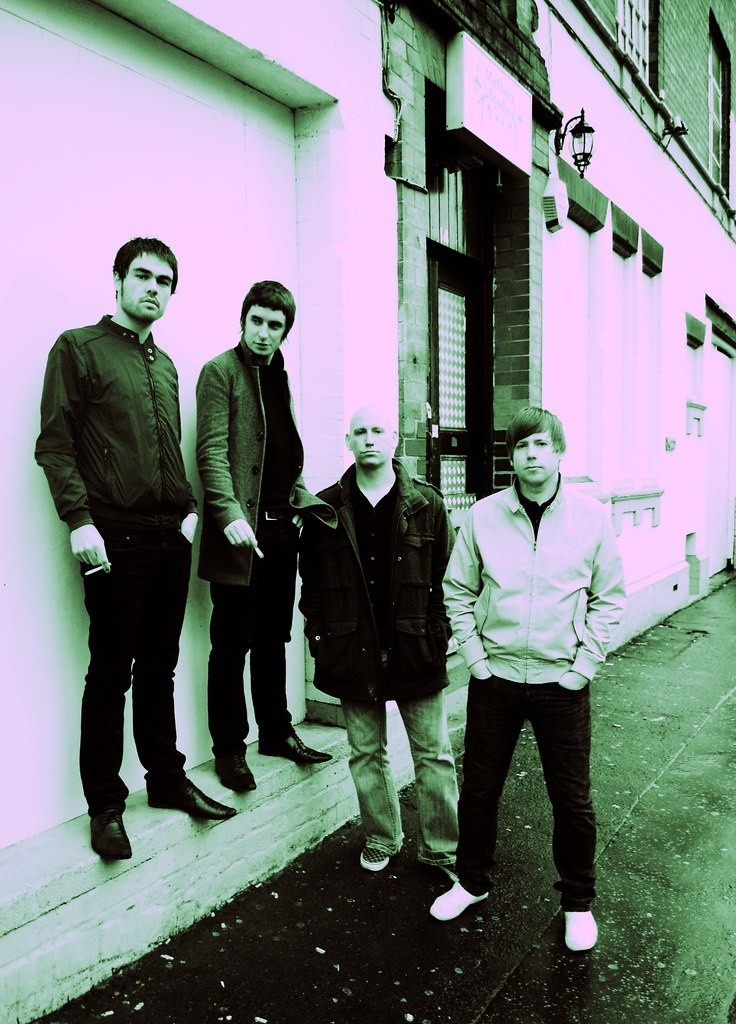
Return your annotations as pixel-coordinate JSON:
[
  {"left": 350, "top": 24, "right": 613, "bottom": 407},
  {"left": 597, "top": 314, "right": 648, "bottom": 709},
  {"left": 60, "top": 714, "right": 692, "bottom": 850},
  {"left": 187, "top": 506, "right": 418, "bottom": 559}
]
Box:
[
  {"left": 215, "top": 754, "right": 256, "bottom": 792},
  {"left": 91, "top": 810, "right": 132, "bottom": 860},
  {"left": 147, "top": 778, "right": 236, "bottom": 819},
  {"left": 258, "top": 730, "right": 333, "bottom": 765}
]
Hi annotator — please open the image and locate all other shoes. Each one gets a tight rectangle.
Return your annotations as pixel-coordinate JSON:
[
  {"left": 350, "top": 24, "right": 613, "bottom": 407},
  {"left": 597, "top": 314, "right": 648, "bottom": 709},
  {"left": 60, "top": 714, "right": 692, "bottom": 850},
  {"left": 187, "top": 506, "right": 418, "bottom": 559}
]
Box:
[
  {"left": 564, "top": 910, "right": 598, "bottom": 951},
  {"left": 429, "top": 881, "right": 488, "bottom": 921},
  {"left": 360, "top": 847, "right": 389, "bottom": 871},
  {"left": 440, "top": 863, "right": 460, "bottom": 882}
]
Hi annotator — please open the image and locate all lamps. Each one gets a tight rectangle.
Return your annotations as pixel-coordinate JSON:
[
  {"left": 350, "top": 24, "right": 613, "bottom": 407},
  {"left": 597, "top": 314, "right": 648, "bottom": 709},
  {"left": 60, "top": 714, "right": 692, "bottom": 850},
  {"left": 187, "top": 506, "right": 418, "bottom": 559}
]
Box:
[
  {"left": 555, "top": 107, "right": 595, "bottom": 179},
  {"left": 662, "top": 116, "right": 689, "bottom": 138}
]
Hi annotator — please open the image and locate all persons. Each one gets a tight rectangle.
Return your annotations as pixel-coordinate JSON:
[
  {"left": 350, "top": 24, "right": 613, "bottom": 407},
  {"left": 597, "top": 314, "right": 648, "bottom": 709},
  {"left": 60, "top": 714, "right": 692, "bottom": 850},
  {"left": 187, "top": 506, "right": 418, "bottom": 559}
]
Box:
[
  {"left": 294, "top": 406, "right": 465, "bottom": 881},
  {"left": 430, "top": 403, "right": 624, "bottom": 952},
  {"left": 197, "top": 280, "right": 339, "bottom": 791},
  {"left": 34, "top": 236, "right": 236, "bottom": 861}
]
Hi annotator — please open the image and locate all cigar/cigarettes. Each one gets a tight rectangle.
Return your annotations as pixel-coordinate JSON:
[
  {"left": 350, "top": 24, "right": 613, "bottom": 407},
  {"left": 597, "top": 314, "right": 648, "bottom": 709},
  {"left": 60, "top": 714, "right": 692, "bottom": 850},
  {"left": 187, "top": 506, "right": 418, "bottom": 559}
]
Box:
[
  {"left": 255, "top": 547, "right": 264, "bottom": 558},
  {"left": 84, "top": 563, "right": 111, "bottom": 576}
]
